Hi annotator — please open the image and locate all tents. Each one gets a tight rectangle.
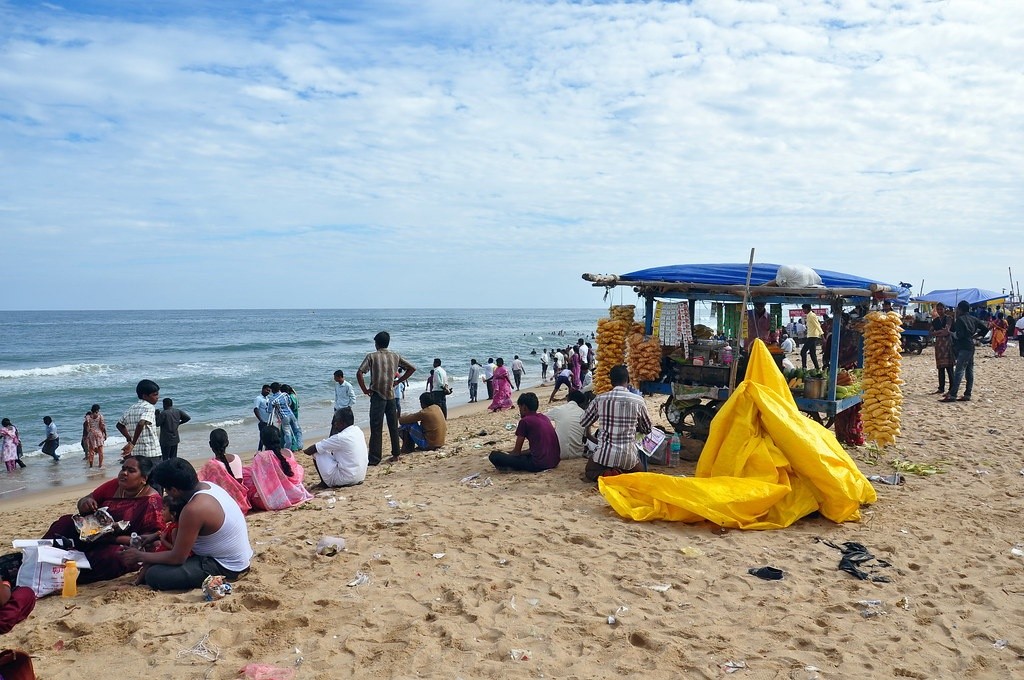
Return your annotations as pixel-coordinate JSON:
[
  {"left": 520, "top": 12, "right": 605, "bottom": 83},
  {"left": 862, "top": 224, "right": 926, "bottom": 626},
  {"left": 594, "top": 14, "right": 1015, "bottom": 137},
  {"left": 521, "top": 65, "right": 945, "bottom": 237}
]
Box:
[{"left": 911, "top": 287, "right": 1010, "bottom": 321}]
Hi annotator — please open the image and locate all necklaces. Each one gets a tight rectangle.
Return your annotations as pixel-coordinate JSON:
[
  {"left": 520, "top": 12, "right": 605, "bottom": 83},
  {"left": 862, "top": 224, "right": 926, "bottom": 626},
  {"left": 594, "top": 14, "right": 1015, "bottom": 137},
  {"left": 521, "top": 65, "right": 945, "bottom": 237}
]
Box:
[{"left": 121, "top": 486, "right": 144, "bottom": 498}]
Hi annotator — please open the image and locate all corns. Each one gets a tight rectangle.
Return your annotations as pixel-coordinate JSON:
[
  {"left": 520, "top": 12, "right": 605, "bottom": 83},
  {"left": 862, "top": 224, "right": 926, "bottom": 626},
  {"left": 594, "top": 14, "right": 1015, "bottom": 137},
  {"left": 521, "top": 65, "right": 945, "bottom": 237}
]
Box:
[{"left": 785, "top": 367, "right": 862, "bottom": 399}]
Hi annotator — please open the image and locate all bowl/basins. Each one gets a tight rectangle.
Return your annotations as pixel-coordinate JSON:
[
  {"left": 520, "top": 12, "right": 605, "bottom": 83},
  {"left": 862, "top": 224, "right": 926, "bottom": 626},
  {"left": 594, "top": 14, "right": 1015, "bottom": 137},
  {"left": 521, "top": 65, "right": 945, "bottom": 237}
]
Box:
[{"left": 698, "top": 339, "right": 724, "bottom": 346}]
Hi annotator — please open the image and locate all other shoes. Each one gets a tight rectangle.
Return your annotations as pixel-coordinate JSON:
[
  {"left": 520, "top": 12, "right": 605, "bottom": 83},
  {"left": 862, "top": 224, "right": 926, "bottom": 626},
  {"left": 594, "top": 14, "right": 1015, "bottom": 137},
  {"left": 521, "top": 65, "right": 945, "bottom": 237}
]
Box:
[
  {"left": 942, "top": 392, "right": 950, "bottom": 397},
  {"left": 956, "top": 395, "right": 970, "bottom": 402},
  {"left": 938, "top": 396, "right": 956, "bottom": 402},
  {"left": 934, "top": 390, "right": 944, "bottom": 394}
]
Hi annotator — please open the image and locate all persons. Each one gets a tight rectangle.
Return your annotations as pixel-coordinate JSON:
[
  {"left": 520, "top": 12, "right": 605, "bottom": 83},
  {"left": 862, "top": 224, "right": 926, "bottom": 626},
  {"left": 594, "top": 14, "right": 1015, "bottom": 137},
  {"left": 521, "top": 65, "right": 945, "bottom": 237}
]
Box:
[
  {"left": 0, "top": 300, "right": 1023, "bottom": 680},
  {"left": 398, "top": 392, "right": 447, "bottom": 454},
  {"left": 489, "top": 392, "right": 561, "bottom": 473},
  {"left": 356, "top": 332, "right": 416, "bottom": 466}
]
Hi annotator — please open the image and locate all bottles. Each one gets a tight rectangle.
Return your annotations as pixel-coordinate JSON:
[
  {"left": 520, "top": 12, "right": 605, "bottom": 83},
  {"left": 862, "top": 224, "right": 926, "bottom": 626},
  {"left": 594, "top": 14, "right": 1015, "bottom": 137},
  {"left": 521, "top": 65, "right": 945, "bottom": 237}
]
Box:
[
  {"left": 63, "top": 560, "right": 77, "bottom": 598},
  {"left": 671, "top": 433, "right": 680, "bottom": 461},
  {"left": 722, "top": 345, "right": 732, "bottom": 365},
  {"left": 129, "top": 532, "right": 146, "bottom": 565}
]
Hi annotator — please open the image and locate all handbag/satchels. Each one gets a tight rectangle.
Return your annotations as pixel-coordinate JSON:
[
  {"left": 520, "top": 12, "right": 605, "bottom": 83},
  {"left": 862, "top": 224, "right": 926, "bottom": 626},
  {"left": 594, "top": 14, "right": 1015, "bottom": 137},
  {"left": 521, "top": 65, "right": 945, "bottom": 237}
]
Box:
[{"left": 15, "top": 533, "right": 91, "bottom": 598}]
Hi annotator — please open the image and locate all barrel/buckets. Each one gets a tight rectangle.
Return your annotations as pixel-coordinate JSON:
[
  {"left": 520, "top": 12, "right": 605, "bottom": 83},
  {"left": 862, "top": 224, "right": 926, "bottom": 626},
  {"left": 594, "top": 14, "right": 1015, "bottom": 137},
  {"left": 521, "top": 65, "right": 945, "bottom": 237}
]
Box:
[{"left": 803, "top": 376, "right": 827, "bottom": 398}]
